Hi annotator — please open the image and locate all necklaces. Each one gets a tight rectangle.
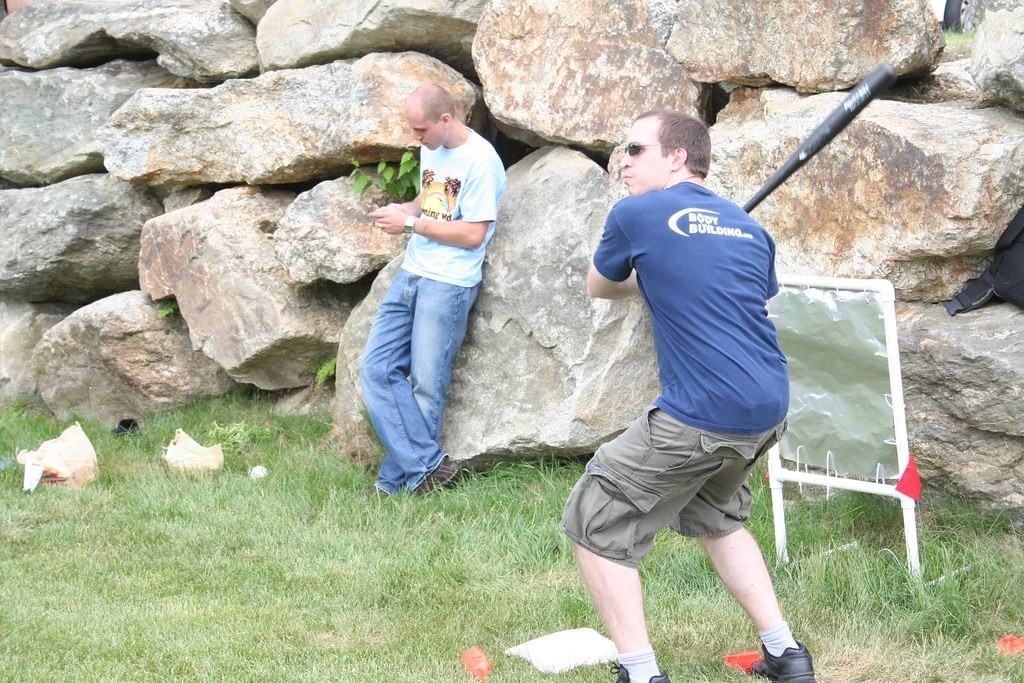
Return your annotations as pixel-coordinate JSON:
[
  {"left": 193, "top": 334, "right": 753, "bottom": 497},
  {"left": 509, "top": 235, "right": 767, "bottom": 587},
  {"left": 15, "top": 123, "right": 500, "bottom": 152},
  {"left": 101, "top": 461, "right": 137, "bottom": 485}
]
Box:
[{"left": 684, "top": 175, "right": 702, "bottom": 182}]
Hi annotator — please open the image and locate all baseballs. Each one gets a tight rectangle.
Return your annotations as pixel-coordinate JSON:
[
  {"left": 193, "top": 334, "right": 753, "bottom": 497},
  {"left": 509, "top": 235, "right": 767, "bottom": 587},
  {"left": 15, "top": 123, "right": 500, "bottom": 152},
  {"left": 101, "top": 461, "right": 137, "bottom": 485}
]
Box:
[{"left": 251, "top": 466, "right": 268, "bottom": 478}]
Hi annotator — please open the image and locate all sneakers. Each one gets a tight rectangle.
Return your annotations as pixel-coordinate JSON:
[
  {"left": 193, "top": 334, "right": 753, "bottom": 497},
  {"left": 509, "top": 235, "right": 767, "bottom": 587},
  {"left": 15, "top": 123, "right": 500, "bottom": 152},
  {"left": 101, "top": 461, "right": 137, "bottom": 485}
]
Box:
[
  {"left": 610, "top": 661, "right": 671, "bottom": 683},
  {"left": 747, "top": 638, "right": 818, "bottom": 683}
]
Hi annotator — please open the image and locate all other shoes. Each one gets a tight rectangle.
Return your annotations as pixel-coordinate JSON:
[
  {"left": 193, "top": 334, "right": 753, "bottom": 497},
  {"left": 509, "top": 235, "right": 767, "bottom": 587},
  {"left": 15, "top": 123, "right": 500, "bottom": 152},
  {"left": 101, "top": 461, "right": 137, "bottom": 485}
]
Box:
[
  {"left": 408, "top": 456, "right": 459, "bottom": 498},
  {"left": 357, "top": 482, "right": 389, "bottom": 500}
]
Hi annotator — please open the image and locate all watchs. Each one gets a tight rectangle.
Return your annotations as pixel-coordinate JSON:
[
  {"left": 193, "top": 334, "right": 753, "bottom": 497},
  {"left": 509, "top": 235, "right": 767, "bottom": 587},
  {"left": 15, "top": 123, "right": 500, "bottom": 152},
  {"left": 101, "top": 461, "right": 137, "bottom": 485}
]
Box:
[{"left": 404, "top": 216, "right": 415, "bottom": 234}]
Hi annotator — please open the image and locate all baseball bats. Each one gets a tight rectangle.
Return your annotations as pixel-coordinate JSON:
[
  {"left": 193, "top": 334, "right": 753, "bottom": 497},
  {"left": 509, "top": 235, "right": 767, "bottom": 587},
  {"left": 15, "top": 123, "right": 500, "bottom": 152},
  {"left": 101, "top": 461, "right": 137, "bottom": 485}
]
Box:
[{"left": 740, "top": 62, "right": 896, "bottom": 214}]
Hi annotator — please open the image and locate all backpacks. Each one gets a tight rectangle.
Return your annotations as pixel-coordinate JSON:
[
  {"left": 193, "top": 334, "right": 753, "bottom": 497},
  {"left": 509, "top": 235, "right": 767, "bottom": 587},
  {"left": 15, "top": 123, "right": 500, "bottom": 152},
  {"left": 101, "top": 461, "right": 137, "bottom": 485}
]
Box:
[{"left": 944, "top": 202, "right": 1024, "bottom": 317}]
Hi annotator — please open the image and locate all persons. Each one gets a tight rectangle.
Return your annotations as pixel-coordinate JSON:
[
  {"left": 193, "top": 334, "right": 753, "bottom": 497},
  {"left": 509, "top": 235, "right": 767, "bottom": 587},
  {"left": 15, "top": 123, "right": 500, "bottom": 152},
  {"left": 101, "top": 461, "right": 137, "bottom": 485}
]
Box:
[
  {"left": 559, "top": 108, "right": 815, "bottom": 683},
  {"left": 359, "top": 85, "right": 507, "bottom": 501}
]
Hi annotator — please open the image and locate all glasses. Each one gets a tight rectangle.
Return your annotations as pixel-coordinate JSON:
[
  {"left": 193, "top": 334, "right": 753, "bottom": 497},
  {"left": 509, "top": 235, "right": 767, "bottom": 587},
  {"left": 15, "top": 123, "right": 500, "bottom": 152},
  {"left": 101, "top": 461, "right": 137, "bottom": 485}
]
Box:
[{"left": 624, "top": 144, "right": 678, "bottom": 156}]
